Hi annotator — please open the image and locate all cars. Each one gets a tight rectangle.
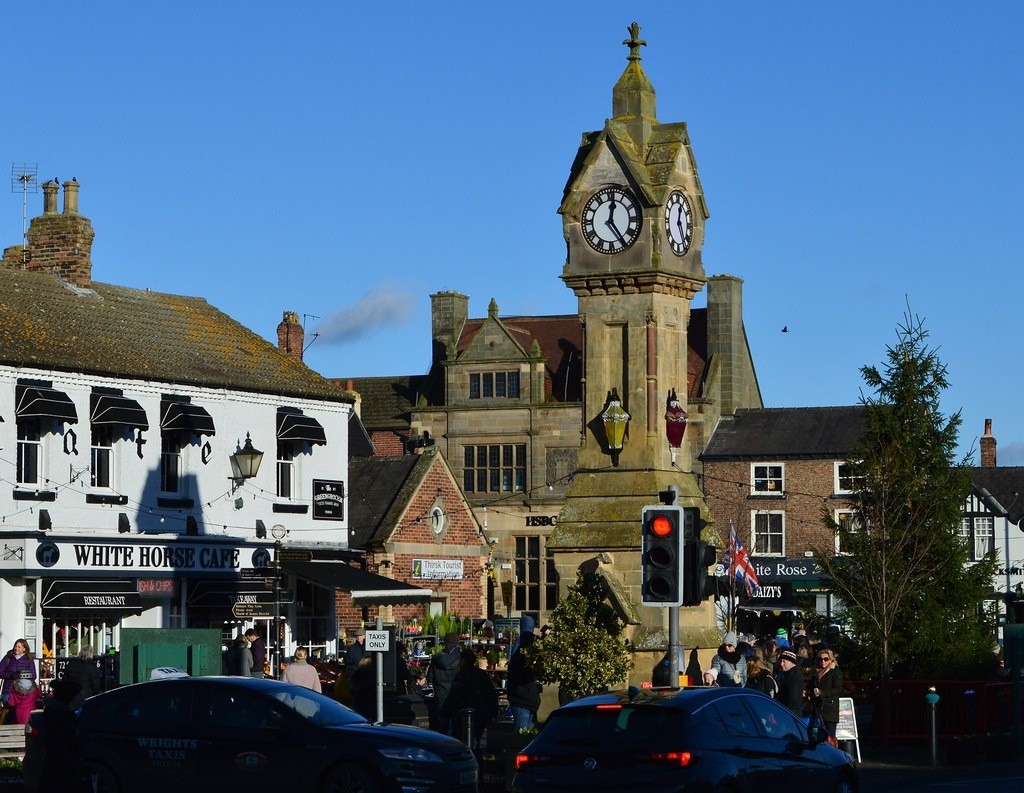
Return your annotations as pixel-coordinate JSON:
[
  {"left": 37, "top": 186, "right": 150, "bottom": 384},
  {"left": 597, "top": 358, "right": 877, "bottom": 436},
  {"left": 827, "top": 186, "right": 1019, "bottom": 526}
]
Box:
[
  {"left": 511, "top": 686, "right": 856, "bottom": 793},
  {"left": 21, "top": 676, "right": 478, "bottom": 793}
]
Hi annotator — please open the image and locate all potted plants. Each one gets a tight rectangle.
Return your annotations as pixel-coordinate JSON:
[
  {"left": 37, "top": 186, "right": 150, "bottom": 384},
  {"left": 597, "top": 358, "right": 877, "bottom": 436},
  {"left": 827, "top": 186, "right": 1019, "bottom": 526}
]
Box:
[
  {"left": 985, "top": 728, "right": 1018, "bottom": 761},
  {"left": 504, "top": 726, "right": 541, "bottom": 793},
  {"left": 395, "top": 610, "right": 518, "bottom": 672},
  {"left": 945, "top": 733, "right": 979, "bottom": 764}
]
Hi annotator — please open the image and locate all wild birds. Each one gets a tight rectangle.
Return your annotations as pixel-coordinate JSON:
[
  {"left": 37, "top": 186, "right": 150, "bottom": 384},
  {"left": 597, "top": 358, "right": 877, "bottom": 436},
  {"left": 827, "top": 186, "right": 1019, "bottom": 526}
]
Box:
[{"left": 781, "top": 326, "right": 790, "bottom": 333}]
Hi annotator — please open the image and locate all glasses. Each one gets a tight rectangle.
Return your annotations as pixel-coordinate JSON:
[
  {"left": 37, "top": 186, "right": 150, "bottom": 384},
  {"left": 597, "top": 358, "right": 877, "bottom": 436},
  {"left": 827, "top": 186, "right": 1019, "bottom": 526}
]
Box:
[
  {"left": 818, "top": 657, "right": 831, "bottom": 661},
  {"left": 723, "top": 644, "right": 733, "bottom": 647}
]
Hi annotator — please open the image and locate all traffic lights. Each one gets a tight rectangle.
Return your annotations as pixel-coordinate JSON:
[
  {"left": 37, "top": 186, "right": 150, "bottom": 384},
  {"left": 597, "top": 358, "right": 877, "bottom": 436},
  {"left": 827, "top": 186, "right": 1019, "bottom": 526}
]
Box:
[
  {"left": 640, "top": 505, "right": 684, "bottom": 608},
  {"left": 682, "top": 507, "right": 718, "bottom": 606}
]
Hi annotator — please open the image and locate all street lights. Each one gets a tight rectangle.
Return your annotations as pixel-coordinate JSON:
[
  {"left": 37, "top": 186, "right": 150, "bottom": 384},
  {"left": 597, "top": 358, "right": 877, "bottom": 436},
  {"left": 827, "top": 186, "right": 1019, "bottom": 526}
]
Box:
[{"left": 924, "top": 685, "right": 940, "bottom": 763}]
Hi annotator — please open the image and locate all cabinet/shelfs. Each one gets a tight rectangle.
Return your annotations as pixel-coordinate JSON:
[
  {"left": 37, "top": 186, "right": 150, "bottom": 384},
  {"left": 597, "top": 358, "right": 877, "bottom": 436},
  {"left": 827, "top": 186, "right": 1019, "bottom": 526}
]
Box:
[{"left": 398, "top": 618, "right": 520, "bottom": 685}]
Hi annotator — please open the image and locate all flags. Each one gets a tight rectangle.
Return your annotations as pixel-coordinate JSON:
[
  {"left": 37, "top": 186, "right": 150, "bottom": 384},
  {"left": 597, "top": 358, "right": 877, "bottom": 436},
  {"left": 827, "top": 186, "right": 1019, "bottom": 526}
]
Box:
[{"left": 724, "top": 526, "right": 758, "bottom": 598}]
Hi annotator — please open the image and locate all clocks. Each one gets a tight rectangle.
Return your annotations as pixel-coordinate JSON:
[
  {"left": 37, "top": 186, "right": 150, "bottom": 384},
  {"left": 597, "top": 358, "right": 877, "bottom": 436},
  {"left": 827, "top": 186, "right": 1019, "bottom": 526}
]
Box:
[
  {"left": 582, "top": 185, "right": 643, "bottom": 255},
  {"left": 664, "top": 190, "right": 694, "bottom": 256}
]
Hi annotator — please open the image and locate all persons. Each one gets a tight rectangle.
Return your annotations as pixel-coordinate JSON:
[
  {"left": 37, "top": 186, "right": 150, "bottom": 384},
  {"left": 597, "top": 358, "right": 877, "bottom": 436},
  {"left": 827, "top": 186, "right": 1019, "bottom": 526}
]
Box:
[
  {"left": 3, "top": 674, "right": 43, "bottom": 753},
  {"left": 986, "top": 634, "right": 1004, "bottom": 671},
  {"left": 651, "top": 650, "right": 671, "bottom": 688},
  {"left": 245, "top": 629, "right": 265, "bottom": 677},
  {"left": 273, "top": 647, "right": 323, "bottom": 721},
  {"left": 0, "top": 639, "right": 36, "bottom": 707},
  {"left": 702, "top": 623, "right": 843, "bottom": 748},
  {"left": 343, "top": 614, "right": 553, "bottom": 750},
  {"left": 59, "top": 645, "right": 120, "bottom": 702},
  {"left": 223, "top": 634, "right": 254, "bottom": 676}
]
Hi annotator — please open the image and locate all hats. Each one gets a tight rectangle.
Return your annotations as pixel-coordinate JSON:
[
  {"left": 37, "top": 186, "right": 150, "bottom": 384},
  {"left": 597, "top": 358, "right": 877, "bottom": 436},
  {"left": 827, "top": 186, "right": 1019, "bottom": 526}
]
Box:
[
  {"left": 354, "top": 629, "right": 366, "bottom": 636},
  {"left": 723, "top": 631, "right": 738, "bottom": 647},
  {"left": 777, "top": 628, "right": 786, "bottom": 635},
  {"left": 777, "top": 650, "right": 798, "bottom": 664},
  {"left": 443, "top": 633, "right": 459, "bottom": 645}
]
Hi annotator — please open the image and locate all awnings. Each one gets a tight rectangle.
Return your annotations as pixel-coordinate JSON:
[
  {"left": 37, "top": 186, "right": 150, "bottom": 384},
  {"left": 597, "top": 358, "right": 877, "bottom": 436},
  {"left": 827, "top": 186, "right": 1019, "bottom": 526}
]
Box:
[
  {"left": 277, "top": 412, "right": 326, "bottom": 446},
  {"left": 277, "top": 561, "right": 432, "bottom": 605},
  {"left": 41, "top": 577, "right": 143, "bottom": 615},
  {"left": 348, "top": 409, "right": 377, "bottom": 465},
  {"left": 90, "top": 394, "right": 148, "bottom": 432},
  {"left": 161, "top": 401, "right": 215, "bottom": 436},
  {"left": 15, "top": 385, "right": 78, "bottom": 425}
]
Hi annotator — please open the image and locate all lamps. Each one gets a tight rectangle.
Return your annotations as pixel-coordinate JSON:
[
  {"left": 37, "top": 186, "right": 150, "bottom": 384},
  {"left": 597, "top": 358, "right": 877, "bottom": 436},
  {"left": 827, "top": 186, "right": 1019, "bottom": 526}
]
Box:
[
  {"left": 666, "top": 388, "right": 690, "bottom": 466},
  {"left": 599, "top": 385, "right": 632, "bottom": 466},
  {"left": 227, "top": 430, "right": 265, "bottom": 478}
]
